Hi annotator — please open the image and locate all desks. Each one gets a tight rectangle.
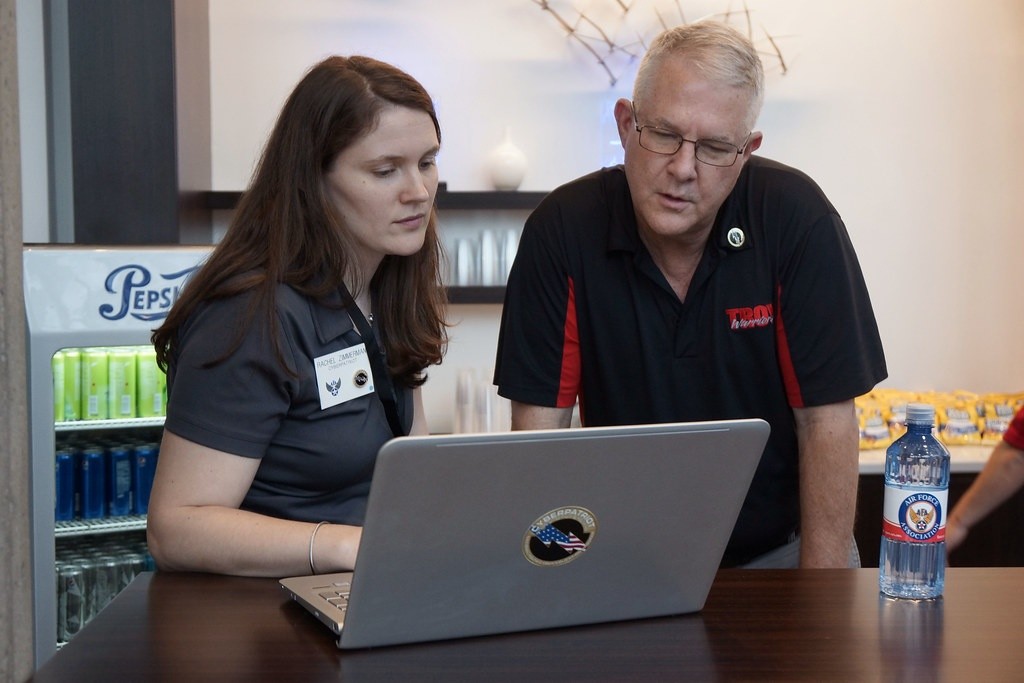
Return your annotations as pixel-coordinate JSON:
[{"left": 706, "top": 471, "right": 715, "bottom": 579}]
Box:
[{"left": 21, "top": 570, "right": 1023, "bottom": 683}]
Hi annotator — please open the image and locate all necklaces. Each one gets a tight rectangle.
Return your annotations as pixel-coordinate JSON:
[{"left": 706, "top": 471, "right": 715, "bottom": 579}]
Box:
[{"left": 367, "top": 292, "right": 375, "bottom": 325}]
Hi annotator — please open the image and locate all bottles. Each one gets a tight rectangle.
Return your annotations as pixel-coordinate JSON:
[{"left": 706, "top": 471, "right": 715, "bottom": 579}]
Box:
[{"left": 875, "top": 406, "right": 951, "bottom": 603}]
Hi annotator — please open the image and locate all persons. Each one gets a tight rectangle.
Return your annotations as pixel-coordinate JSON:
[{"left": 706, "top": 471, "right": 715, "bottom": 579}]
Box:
[
  {"left": 144, "top": 53, "right": 464, "bottom": 578},
  {"left": 489, "top": 20, "right": 893, "bottom": 571},
  {"left": 945, "top": 402, "right": 1024, "bottom": 574}
]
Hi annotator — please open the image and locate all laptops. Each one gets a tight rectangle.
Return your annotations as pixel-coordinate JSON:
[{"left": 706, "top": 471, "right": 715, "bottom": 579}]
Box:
[{"left": 277, "top": 418, "right": 771, "bottom": 650}]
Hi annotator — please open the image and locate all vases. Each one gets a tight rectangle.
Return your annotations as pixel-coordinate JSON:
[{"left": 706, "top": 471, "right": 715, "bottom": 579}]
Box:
[{"left": 490, "top": 123, "right": 528, "bottom": 191}]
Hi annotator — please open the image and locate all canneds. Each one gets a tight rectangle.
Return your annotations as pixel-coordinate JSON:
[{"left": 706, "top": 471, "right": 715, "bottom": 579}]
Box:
[
  {"left": 53, "top": 348, "right": 168, "bottom": 420},
  {"left": 55, "top": 428, "right": 162, "bottom": 522},
  {"left": 56, "top": 529, "right": 153, "bottom": 643}
]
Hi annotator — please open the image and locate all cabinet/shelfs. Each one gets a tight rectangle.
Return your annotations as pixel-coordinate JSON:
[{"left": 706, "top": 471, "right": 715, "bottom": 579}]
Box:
[{"left": 174, "top": 188, "right": 557, "bottom": 305}]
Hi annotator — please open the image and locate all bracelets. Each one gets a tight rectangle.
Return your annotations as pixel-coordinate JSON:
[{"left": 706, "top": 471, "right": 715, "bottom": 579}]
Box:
[{"left": 310, "top": 521, "right": 333, "bottom": 578}]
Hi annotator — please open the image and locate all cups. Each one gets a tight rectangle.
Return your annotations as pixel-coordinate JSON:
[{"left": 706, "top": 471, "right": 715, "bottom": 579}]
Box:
[
  {"left": 438, "top": 225, "right": 523, "bottom": 288},
  {"left": 452, "top": 361, "right": 508, "bottom": 432}
]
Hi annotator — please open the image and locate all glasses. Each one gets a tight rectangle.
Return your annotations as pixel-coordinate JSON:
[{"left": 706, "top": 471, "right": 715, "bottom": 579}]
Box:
[{"left": 632, "top": 100, "right": 751, "bottom": 167}]
